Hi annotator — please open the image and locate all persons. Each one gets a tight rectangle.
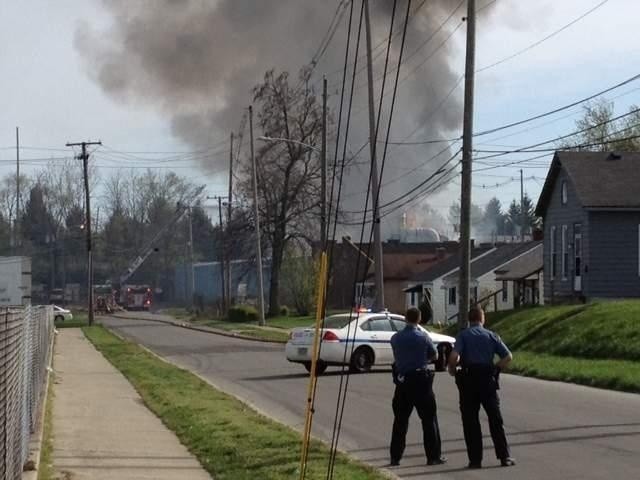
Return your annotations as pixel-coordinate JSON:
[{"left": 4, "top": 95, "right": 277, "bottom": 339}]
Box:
[
  {"left": 389, "top": 305, "right": 450, "bottom": 466},
  {"left": 447, "top": 307, "right": 518, "bottom": 468}
]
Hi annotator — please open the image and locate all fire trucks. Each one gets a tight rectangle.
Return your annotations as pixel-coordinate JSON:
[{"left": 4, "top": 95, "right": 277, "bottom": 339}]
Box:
[{"left": 113, "top": 183, "right": 213, "bottom": 312}]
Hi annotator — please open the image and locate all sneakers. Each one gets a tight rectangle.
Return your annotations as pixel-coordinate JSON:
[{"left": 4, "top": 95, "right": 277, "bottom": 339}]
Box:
[
  {"left": 427, "top": 457, "right": 448, "bottom": 466},
  {"left": 500, "top": 458, "right": 517, "bottom": 466}
]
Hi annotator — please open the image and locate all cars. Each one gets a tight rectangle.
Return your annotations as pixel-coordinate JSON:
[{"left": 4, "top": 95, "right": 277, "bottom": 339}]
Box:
[
  {"left": 27, "top": 288, "right": 72, "bottom": 322},
  {"left": 282, "top": 306, "right": 456, "bottom": 378}
]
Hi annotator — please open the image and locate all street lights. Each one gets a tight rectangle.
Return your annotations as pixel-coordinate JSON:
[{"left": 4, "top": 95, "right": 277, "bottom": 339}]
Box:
[{"left": 252, "top": 136, "right": 329, "bottom": 322}]
